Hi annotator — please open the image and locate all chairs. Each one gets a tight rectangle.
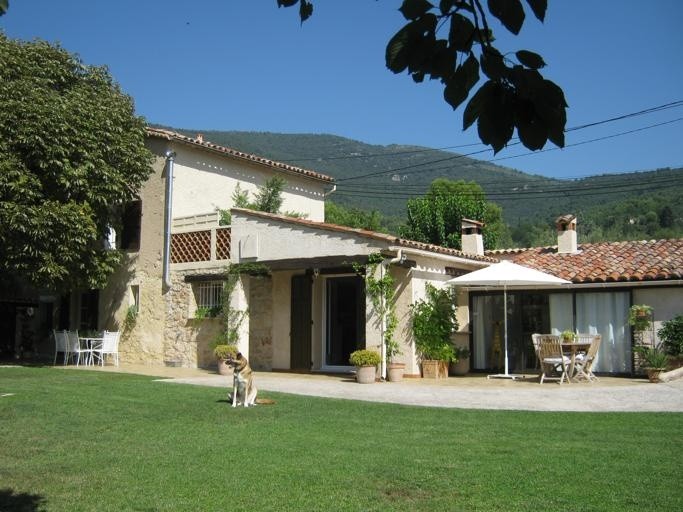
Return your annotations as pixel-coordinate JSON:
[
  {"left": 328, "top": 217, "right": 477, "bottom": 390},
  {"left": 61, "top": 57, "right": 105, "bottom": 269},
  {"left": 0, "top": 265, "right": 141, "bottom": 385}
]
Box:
[
  {"left": 51, "top": 328, "right": 120, "bottom": 368},
  {"left": 530, "top": 326, "right": 601, "bottom": 386}
]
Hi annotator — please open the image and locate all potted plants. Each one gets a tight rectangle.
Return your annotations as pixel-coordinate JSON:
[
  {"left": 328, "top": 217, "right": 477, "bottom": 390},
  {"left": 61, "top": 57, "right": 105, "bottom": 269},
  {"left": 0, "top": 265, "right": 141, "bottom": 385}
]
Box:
[
  {"left": 632, "top": 345, "right": 669, "bottom": 383},
  {"left": 628, "top": 305, "right": 654, "bottom": 332},
  {"left": 407, "top": 282, "right": 471, "bottom": 378},
  {"left": 213, "top": 345, "right": 238, "bottom": 375},
  {"left": 349, "top": 350, "right": 381, "bottom": 384}
]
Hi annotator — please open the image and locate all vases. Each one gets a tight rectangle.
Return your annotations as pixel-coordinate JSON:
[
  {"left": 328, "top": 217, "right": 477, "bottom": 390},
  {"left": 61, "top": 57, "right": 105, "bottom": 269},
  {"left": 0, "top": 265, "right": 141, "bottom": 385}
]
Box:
[{"left": 387, "top": 363, "right": 406, "bottom": 382}]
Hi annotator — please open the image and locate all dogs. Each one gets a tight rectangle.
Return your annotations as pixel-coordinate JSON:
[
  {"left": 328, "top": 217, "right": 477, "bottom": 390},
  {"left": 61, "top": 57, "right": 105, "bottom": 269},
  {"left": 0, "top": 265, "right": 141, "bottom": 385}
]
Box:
[{"left": 225, "top": 351, "right": 276, "bottom": 409}]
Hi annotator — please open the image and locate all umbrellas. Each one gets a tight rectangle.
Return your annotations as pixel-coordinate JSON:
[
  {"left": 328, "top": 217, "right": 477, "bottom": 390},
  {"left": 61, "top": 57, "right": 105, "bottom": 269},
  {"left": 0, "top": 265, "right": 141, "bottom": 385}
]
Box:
[{"left": 440, "top": 257, "right": 573, "bottom": 375}]
